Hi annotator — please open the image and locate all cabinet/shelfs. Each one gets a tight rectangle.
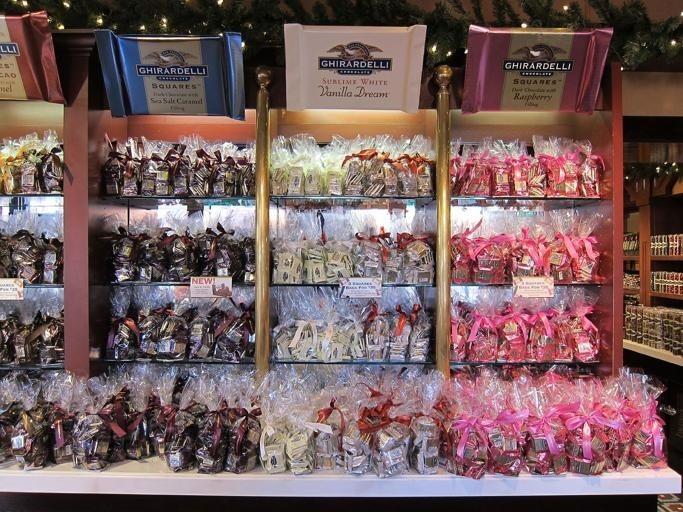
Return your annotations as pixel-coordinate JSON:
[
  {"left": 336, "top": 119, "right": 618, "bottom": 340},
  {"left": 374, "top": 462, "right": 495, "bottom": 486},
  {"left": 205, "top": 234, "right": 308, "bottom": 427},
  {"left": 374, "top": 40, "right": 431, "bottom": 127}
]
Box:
[
  {"left": 623, "top": 172, "right": 683, "bottom": 366},
  {"left": 0, "top": 25, "right": 683, "bottom": 500}
]
